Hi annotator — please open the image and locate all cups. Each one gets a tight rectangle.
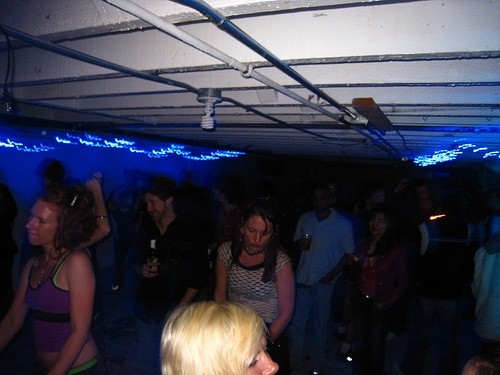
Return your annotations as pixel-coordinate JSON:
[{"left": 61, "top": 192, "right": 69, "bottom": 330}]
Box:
[{"left": 303, "top": 232, "right": 311, "bottom": 251}]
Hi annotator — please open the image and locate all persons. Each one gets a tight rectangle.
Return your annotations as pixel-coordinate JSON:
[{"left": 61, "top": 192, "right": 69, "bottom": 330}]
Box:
[
  {"left": 0, "top": 180, "right": 109, "bottom": 374},
  {"left": 0, "top": 159, "right": 110, "bottom": 323},
  {"left": 135, "top": 162, "right": 500, "bottom": 375}
]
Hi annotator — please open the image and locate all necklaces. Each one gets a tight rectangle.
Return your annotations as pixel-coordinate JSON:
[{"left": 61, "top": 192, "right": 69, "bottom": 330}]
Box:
[
  {"left": 162, "top": 300, "right": 279, "bottom": 375},
  {"left": 37, "top": 248, "right": 69, "bottom": 284},
  {"left": 245, "top": 247, "right": 266, "bottom": 257}
]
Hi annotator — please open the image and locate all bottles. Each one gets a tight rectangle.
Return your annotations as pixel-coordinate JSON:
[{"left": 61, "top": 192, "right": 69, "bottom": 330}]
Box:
[
  {"left": 148, "top": 240, "right": 158, "bottom": 274},
  {"left": 67, "top": 192, "right": 79, "bottom": 211},
  {"left": 207, "top": 248, "right": 213, "bottom": 269},
  {"left": 79, "top": 172, "right": 103, "bottom": 197}
]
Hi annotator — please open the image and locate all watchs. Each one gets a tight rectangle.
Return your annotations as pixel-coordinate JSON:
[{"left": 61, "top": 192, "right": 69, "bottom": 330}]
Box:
[{"left": 393, "top": 186, "right": 401, "bottom": 195}]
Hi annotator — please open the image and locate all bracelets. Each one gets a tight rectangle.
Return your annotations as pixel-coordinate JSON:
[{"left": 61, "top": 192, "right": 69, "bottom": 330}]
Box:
[{"left": 97, "top": 216, "right": 109, "bottom": 222}]
